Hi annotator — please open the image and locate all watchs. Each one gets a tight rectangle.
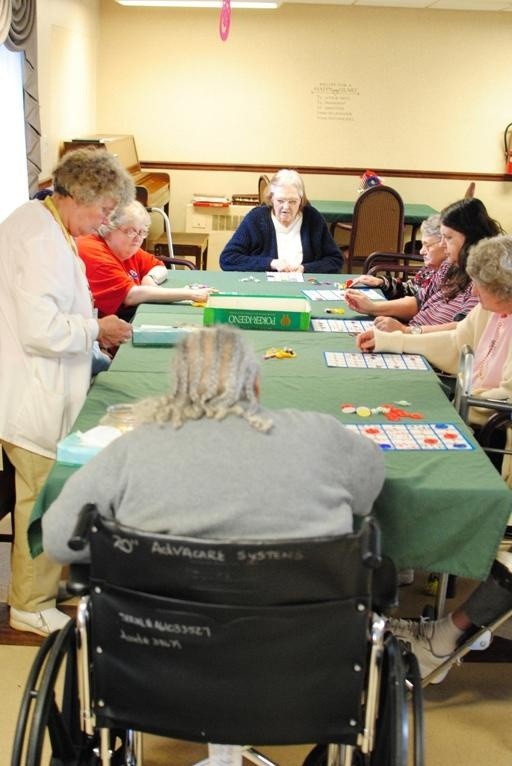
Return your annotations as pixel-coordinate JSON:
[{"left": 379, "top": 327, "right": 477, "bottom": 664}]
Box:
[
  {"left": 411, "top": 326, "right": 423, "bottom": 335},
  {"left": 145, "top": 274, "right": 156, "bottom": 283}
]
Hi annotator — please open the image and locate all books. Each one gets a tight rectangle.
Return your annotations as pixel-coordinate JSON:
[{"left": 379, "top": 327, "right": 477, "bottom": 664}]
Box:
[{"left": 192, "top": 194, "right": 231, "bottom": 208}]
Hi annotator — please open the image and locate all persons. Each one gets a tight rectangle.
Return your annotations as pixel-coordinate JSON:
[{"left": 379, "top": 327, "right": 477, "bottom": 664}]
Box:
[
  {"left": 345, "top": 214, "right": 450, "bottom": 301},
  {"left": 356, "top": 233, "right": 511, "bottom": 476},
  {"left": 34, "top": 141, "right": 106, "bottom": 198},
  {"left": 0, "top": 146, "right": 134, "bottom": 638},
  {"left": 220, "top": 169, "right": 343, "bottom": 274},
  {"left": 370, "top": 558, "right": 512, "bottom": 686},
  {"left": 41, "top": 325, "right": 385, "bottom": 565},
  {"left": 343, "top": 199, "right": 508, "bottom": 334},
  {"left": 74, "top": 199, "right": 219, "bottom": 318}
]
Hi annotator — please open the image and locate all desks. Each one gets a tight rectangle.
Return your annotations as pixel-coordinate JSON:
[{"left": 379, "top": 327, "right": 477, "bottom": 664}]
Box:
[
  {"left": 308, "top": 197, "right": 445, "bottom": 255},
  {"left": 34, "top": 260, "right": 509, "bottom": 627}
]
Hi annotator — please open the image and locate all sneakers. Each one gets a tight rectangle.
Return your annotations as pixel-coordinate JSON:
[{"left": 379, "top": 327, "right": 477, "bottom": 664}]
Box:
[{"left": 369, "top": 612, "right": 491, "bottom": 685}]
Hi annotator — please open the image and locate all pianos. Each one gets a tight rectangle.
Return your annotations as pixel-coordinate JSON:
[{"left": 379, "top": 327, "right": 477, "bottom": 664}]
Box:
[{"left": 63, "top": 134, "right": 171, "bottom": 250}]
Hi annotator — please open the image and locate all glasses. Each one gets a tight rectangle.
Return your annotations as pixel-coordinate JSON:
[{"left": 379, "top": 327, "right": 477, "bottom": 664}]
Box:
[{"left": 119, "top": 227, "right": 150, "bottom": 239}]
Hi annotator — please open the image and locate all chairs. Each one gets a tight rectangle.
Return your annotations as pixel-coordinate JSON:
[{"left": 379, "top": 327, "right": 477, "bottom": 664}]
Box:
[{"left": 326, "top": 185, "right": 405, "bottom": 274}]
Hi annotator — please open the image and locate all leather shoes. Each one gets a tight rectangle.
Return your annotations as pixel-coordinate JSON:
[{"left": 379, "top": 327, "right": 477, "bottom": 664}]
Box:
[{"left": 9, "top": 606, "right": 74, "bottom": 638}]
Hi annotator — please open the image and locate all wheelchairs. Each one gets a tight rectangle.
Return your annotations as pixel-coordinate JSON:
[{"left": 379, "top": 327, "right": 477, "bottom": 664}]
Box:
[{"left": 11, "top": 503, "right": 425, "bottom": 766}]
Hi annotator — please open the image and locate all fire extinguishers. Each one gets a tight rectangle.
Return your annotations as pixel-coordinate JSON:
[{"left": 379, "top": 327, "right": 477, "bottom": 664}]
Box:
[{"left": 504, "top": 123, "right": 512, "bottom": 175}]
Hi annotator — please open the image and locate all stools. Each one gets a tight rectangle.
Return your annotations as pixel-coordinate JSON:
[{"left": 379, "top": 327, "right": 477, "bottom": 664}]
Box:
[{"left": 156, "top": 231, "right": 209, "bottom": 270}]
